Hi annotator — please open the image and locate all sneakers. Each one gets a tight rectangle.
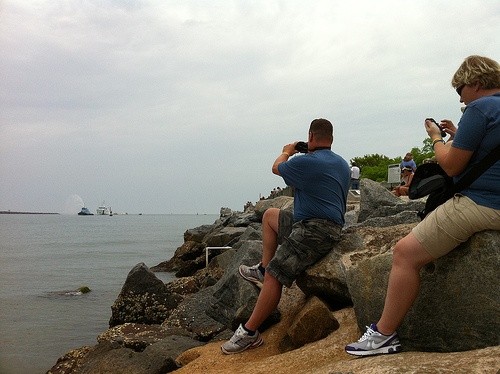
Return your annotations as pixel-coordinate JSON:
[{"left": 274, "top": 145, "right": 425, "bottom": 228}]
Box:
[
  {"left": 345, "top": 324, "right": 402, "bottom": 355},
  {"left": 239, "top": 262, "right": 265, "bottom": 288},
  {"left": 221, "top": 323, "right": 263, "bottom": 354}
]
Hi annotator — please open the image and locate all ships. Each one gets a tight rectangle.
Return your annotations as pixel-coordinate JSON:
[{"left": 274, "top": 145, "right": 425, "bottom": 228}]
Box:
[
  {"left": 96, "top": 206, "right": 109, "bottom": 215},
  {"left": 78, "top": 207, "right": 94, "bottom": 215}
]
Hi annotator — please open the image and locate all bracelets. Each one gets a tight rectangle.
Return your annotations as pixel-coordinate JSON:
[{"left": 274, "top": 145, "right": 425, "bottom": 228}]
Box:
[
  {"left": 432, "top": 139, "right": 446, "bottom": 149},
  {"left": 282, "top": 152, "right": 289, "bottom": 157}
]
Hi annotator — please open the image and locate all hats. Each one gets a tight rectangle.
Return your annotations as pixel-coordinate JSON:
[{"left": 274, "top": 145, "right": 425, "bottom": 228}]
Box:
[{"left": 402, "top": 165, "right": 413, "bottom": 172}]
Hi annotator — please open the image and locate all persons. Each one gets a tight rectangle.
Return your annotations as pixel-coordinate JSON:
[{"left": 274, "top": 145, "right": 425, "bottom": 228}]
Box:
[
  {"left": 344, "top": 55, "right": 500, "bottom": 356},
  {"left": 350, "top": 162, "right": 361, "bottom": 190},
  {"left": 221, "top": 118, "right": 351, "bottom": 354},
  {"left": 401, "top": 153, "right": 417, "bottom": 183},
  {"left": 392, "top": 166, "right": 415, "bottom": 196}
]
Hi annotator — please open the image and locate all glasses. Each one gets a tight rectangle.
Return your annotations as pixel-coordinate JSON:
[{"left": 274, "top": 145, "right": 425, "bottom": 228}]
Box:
[{"left": 456, "top": 83, "right": 466, "bottom": 96}]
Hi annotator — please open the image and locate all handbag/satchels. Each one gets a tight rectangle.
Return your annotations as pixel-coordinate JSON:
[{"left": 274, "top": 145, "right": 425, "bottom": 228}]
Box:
[{"left": 408, "top": 160, "right": 455, "bottom": 221}]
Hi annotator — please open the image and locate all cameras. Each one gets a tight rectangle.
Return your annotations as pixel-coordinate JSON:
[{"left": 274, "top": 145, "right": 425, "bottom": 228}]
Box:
[{"left": 295, "top": 142, "right": 308, "bottom": 153}]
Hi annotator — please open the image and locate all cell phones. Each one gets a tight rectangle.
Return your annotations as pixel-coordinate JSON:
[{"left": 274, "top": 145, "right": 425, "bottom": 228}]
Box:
[{"left": 425, "top": 118, "right": 446, "bottom": 137}]
